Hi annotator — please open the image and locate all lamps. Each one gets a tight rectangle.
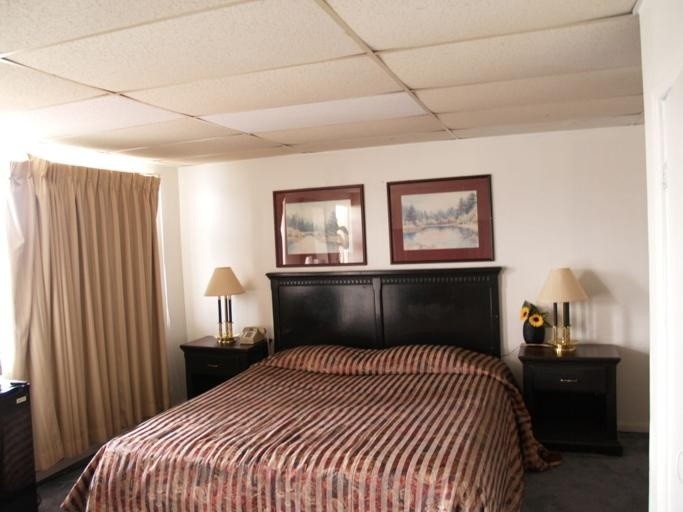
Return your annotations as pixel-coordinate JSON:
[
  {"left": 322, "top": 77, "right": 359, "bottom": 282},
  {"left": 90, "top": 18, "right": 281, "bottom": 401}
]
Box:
[
  {"left": 538, "top": 268, "right": 588, "bottom": 352},
  {"left": 204, "top": 267, "right": 245, "bottom": 344}
]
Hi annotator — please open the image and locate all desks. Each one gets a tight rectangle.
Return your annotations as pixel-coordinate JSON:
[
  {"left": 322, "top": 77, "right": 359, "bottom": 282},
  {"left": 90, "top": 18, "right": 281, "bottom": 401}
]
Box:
[{"left": 0, "top": 378, "right": 38, "bottom": 512}]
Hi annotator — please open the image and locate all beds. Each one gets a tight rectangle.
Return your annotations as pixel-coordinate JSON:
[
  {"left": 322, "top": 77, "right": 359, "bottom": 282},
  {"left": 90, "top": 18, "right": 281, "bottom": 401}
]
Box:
[{"left": 56, "top": 267, "right": 561, "bottom": 512}]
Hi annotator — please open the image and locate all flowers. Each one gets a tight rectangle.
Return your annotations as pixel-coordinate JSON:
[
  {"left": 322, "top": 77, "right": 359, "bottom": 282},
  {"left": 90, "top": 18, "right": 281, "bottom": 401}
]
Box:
[{"left": 519, "top": 300, "right": 553, "bottom": 327}]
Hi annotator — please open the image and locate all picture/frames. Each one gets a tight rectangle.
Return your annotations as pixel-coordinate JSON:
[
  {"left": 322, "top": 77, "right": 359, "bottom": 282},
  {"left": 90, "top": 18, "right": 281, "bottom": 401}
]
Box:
[
  {"left": 386, "top": 174, "right": 494, "bottom": 264},
  {"left": 272, "top": 185, "right": 367, "bottom": 267}
]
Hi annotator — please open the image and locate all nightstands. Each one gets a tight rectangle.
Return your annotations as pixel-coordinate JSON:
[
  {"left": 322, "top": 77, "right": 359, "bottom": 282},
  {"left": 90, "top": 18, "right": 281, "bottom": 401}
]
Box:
[
  {"left": 518, "top": 344, "right": 623, "bottom": 457},
  {"left": 180, "top": 336, "right": 273, "bottom": 400}
]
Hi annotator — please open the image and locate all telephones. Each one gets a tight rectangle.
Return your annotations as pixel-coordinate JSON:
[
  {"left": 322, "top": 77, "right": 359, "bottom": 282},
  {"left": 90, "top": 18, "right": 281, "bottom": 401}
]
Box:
[{"left": 239, "top": 326, "right": 267, "bottom": 344}]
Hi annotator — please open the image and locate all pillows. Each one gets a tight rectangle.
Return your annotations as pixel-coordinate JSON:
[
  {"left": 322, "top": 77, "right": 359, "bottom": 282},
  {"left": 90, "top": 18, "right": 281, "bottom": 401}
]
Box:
[{"left": 249, "top": 345, "right": 561, "bottom": 472}]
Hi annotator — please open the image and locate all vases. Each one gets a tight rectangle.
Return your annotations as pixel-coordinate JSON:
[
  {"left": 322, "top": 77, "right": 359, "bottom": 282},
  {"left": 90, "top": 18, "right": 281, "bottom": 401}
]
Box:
[{"left": 523, "top": 319, "right": 545, "bottom": 344}]
